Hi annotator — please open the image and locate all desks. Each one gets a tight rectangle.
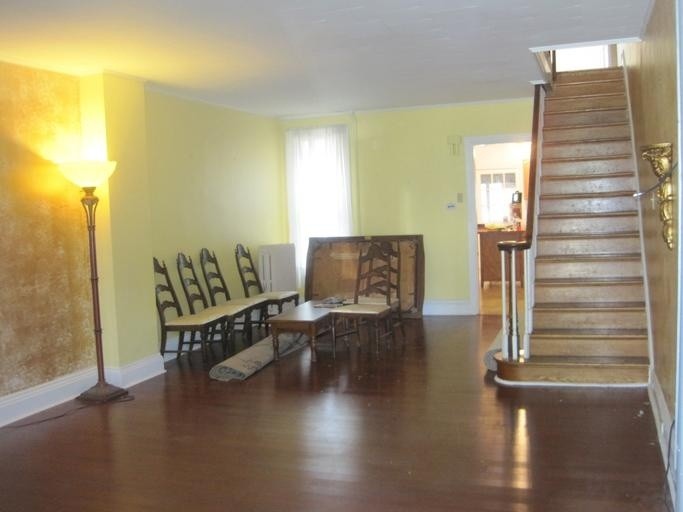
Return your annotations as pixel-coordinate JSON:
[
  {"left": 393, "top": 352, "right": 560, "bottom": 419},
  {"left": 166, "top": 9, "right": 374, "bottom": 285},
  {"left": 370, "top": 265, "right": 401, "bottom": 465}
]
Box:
[{"left": 273, "top": 295, "right": 347, "bottom": 360}]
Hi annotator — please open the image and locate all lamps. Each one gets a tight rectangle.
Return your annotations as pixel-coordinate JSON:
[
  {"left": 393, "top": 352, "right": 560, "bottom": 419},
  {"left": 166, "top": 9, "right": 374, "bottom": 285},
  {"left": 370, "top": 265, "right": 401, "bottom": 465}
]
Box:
[{"left": 69, "top": 162, "right": 140, "bottom": 403}]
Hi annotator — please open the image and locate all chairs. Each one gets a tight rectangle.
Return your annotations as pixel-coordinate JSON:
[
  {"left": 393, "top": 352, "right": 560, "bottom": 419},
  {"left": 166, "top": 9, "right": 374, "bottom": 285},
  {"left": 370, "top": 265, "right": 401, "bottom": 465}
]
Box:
[
  {"left": 173, "top": 253, "right": 250, "bottom": 348},
  {"left": 152, "top": 259, "right": 227, "bottom": 366},
  {"left": 363, "top": 244, "right": 406, "bottom": 348},
  {"left": 202, "top": 250, "right": 268, "bottom": 342},
  {"left": 232, "top": 241, "right": 295, "bottom": 330},
  {"left": 338, "top": 242, "right": 391, "bottom": 359}
]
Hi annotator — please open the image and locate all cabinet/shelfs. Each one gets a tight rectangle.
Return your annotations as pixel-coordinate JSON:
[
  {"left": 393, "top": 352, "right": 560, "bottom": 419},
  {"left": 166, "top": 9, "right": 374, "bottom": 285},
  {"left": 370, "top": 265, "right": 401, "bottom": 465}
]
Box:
[{"left": 478, "top": 223, "right": 524, "bottom": 290}]
